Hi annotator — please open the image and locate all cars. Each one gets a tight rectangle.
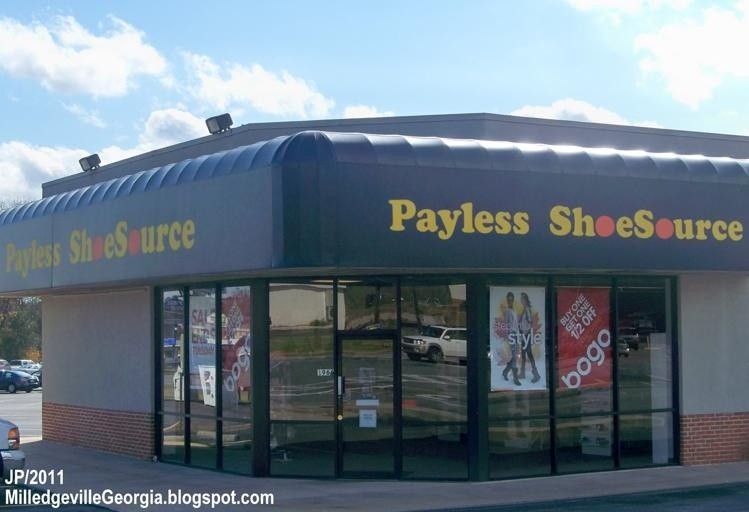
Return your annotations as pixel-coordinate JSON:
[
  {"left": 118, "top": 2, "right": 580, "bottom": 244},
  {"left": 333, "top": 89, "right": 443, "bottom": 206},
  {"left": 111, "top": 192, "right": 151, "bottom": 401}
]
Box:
[
  {"left": 401, "top": 325, "right": 468, "bottom": 365},
  {"left": 0, "top": 359, "right": 42, "bottom": 393},
  {"left": 616, "top": 313, "right": 654, "bottom": 357},
  {"left": 0, "top": 419, "right": 26, "bottom": 485}
]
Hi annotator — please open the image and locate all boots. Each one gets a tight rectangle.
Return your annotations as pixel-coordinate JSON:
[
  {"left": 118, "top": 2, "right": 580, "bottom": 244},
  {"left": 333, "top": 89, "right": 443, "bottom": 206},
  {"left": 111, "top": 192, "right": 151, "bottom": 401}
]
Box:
[
  {"left": 512, "top": 367, "right": 526, "bottom": 386},
  {"left": 531, "top": 368, "right": 541, "bottom": 383},
  {"left": 501, "top": 363, "right": 510, "bottom": 381}
]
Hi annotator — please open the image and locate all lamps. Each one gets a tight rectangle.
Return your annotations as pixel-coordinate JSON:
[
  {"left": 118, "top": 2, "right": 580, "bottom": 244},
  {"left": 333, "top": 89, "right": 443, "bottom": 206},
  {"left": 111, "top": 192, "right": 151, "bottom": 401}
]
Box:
[
  {"left": 79, "top": 154, "right": 101, "bottom": 172},
  {"left": 206, "top": 113, "right": 233, "bottom": 135}
]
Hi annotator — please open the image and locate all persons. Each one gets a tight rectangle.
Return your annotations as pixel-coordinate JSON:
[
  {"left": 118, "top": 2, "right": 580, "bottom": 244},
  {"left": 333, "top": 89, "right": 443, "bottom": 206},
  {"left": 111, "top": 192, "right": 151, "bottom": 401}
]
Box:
[
  {"left": 501, "top": 292, "right": 520, "bottom": 385},
  {"left": 517, "top": 292, "right": 540, "bottom": 383}
]
